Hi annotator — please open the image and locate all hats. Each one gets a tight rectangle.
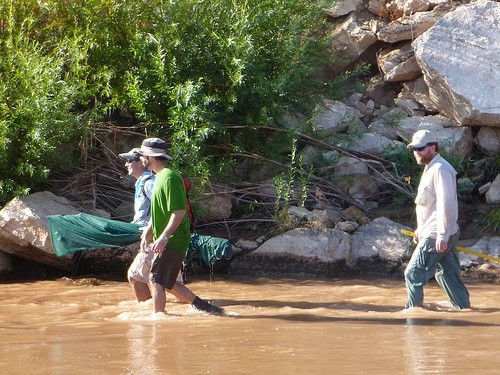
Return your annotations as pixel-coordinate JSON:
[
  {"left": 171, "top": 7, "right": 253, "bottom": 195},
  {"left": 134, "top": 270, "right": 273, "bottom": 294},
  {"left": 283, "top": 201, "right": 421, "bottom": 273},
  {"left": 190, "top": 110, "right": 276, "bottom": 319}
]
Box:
[
  {"left": 407, "top": 129, "right": 437, "bottom": 148},
  {"left": 119, "top": 148, "right": 143, "bottom": 161},
  {"left": 136, "top": 138, "right": 173, "bottom": 160}
]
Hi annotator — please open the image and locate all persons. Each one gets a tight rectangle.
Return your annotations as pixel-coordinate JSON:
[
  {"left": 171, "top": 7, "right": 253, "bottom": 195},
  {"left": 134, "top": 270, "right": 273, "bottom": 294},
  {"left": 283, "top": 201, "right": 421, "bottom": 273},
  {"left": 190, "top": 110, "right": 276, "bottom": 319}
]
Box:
[
  {"left": 138, "top": 137, "right": 224, "bottom": 318},
  {"left": 404, "top": 130, "right": 471, "bottom": 312},
  {"left": 124, "top": 147, "right": 187, "bottom": 304}
]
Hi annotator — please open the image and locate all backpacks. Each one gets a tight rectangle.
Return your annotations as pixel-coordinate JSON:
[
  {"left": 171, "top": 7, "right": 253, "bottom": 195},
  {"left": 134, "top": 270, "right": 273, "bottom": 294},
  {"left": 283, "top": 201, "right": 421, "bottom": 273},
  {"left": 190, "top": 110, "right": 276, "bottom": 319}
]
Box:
[{"left": 140, "top": 172, "right": 194, "bottom": 230}]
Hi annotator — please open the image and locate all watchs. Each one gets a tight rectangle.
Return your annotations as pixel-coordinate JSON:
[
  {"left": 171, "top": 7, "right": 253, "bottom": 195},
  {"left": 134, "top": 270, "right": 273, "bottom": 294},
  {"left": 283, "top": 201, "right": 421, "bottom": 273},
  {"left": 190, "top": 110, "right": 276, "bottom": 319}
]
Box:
[{"left": 160, "top": 235, "right": 168, "bottom": 242}]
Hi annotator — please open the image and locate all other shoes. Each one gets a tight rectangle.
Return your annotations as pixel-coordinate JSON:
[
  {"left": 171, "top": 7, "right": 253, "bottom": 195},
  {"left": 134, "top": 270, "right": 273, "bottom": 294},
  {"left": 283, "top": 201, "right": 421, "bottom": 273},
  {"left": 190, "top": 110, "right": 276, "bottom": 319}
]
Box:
[{"left": 207, "top": 305, "right": 224, "bottom": 317}]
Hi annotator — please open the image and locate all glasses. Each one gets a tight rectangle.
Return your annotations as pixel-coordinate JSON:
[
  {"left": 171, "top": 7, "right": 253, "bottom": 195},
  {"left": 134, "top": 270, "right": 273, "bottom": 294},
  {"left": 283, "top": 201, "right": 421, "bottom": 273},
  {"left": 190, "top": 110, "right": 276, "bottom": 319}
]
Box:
[
  {"left": 126, "top": 159, "right": 139, "bottom": 163},
  {"left": 412, "top": 146, "right": 429, "bottom": 151}
]
